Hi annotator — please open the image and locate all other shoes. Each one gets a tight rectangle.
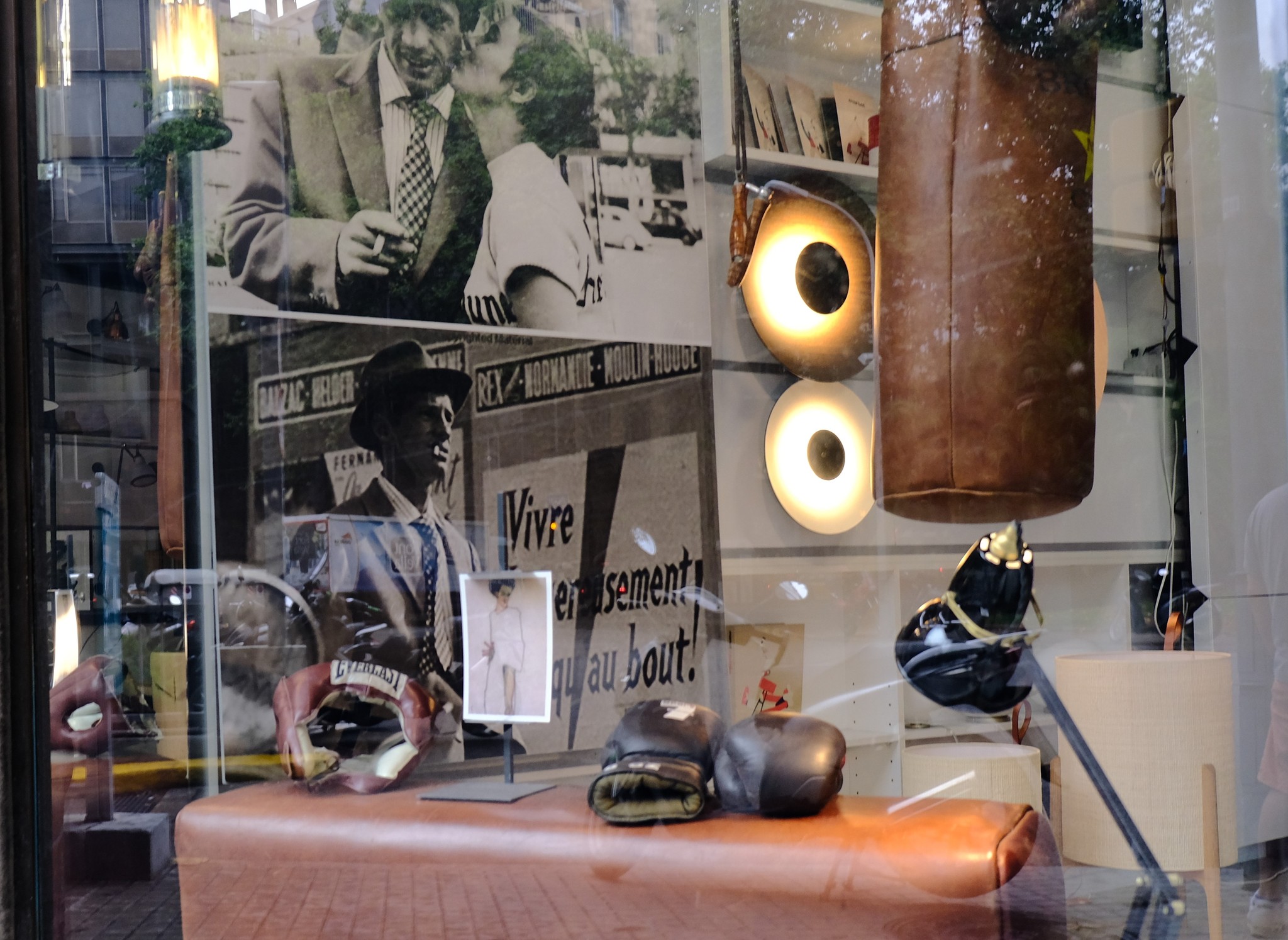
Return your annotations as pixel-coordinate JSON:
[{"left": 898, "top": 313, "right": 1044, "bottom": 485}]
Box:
[{"left": 1248, "top": 886, "right": 1288, "bottom": 938}]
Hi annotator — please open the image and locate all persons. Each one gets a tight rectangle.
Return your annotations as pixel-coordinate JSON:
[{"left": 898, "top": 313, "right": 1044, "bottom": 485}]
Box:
[
  {"left": 212, "top": 1, "right": 619, "bottom": 343},
  {"left": 283, "top": 337, "right": 481, "bottom": 724},
  {"left": 483, "top": 580, "right": 527, "bottom": 720}
]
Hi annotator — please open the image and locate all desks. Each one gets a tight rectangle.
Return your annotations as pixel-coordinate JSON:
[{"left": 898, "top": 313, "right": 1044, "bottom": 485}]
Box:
[{"left": 177, "top": 783, "right": 1065, "bottom": 940}]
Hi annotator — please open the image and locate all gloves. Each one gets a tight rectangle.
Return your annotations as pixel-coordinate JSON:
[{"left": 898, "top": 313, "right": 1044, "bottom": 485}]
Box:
[
  {"left": 714, "top": 710, "right": 848, "bottom": 819},
  {"left": 587, "top": 698, "right": 726, "bottom": 824}
]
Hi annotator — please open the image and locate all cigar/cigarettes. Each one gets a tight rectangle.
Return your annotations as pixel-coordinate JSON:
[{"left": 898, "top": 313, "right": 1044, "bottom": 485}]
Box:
[{"left": 372, "top": 233, "right": 386, "bottom": 261}]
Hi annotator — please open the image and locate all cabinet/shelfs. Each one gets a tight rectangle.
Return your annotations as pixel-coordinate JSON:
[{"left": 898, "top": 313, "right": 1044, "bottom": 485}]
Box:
[{"left": 696, "top": 0, "right": 1183, "bottom": 799}]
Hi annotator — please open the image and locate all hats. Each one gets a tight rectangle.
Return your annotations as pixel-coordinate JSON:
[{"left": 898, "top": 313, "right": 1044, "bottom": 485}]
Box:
[{"left": 349, "top": 339, "right": 473, "bottom": 452}]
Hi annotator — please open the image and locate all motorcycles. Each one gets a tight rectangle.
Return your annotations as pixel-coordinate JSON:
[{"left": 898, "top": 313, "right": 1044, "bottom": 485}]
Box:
[{"left": 230, "top": 551, "right": 514, "bottom": 755}]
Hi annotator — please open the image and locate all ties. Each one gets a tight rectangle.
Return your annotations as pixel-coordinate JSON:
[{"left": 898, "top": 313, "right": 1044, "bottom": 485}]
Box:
[
  {"left": 393, "top": 103, "right": 438, "bottom": 279},
  {"left": 419, "top": 518, "right": 455, "bottom": 673},
  {"left": 416, "top": 515, "right": 437, "bottom": 679}
]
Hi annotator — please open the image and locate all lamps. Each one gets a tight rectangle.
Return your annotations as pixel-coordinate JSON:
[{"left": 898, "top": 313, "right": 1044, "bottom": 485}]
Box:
[
  {"left": 894, "top": 518, "right": 1187, "bottom": 939},
  {"left": 145, "top": 0, "right": 233, "bottom": 151},
  {"left": 730, "top": 0, "right": 876, "bottom": 534}
]
